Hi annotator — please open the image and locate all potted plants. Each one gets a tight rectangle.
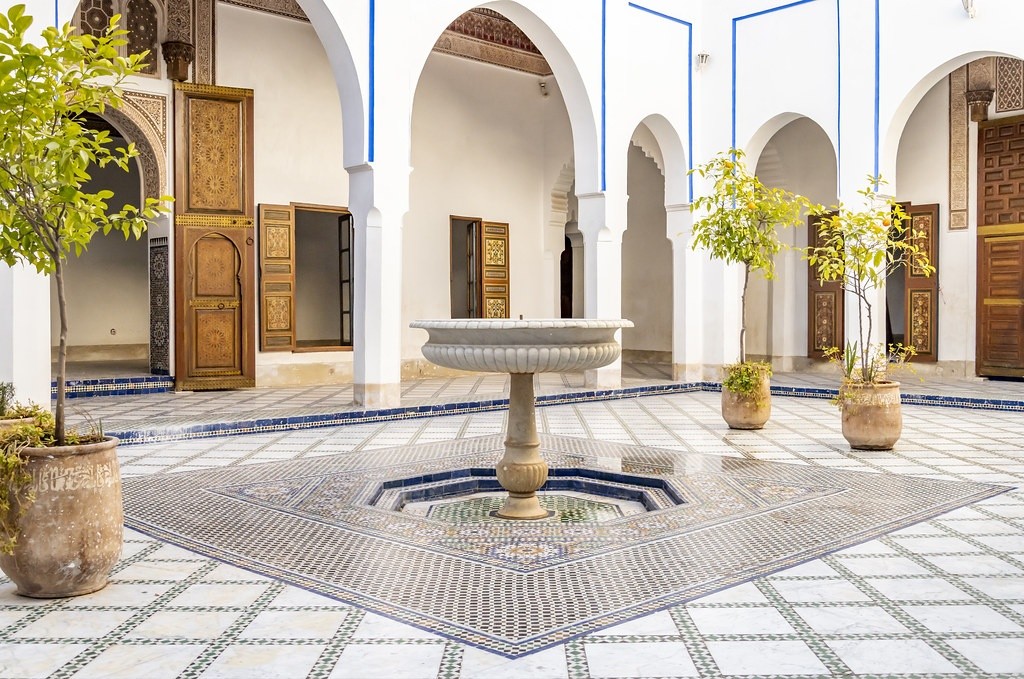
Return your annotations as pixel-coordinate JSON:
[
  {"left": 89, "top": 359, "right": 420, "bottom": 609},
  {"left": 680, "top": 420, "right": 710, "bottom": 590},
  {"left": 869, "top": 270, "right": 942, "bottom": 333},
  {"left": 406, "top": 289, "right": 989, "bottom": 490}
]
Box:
[
  {"left": 0, "top": 5, "right": 176, "bottom": 598},
  {"left": 803, "top": 174, "right": 936, "bottom": 451},
  {"left": 0, "top": 382, "right": 41, "bottom": 426},
  {"left": 687, "top": 148, "right": 802, "bottom": 429}
]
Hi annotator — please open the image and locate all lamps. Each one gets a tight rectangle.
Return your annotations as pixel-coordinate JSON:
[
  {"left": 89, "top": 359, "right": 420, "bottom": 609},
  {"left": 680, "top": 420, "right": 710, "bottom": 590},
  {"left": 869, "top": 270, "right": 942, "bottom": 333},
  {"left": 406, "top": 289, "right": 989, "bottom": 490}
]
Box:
[
  {"left": 962, "top": 0, "right": 976, "bottom": 18},
  {"left": 698, "top": 50, "right": 709, "bottom": 67}
]
